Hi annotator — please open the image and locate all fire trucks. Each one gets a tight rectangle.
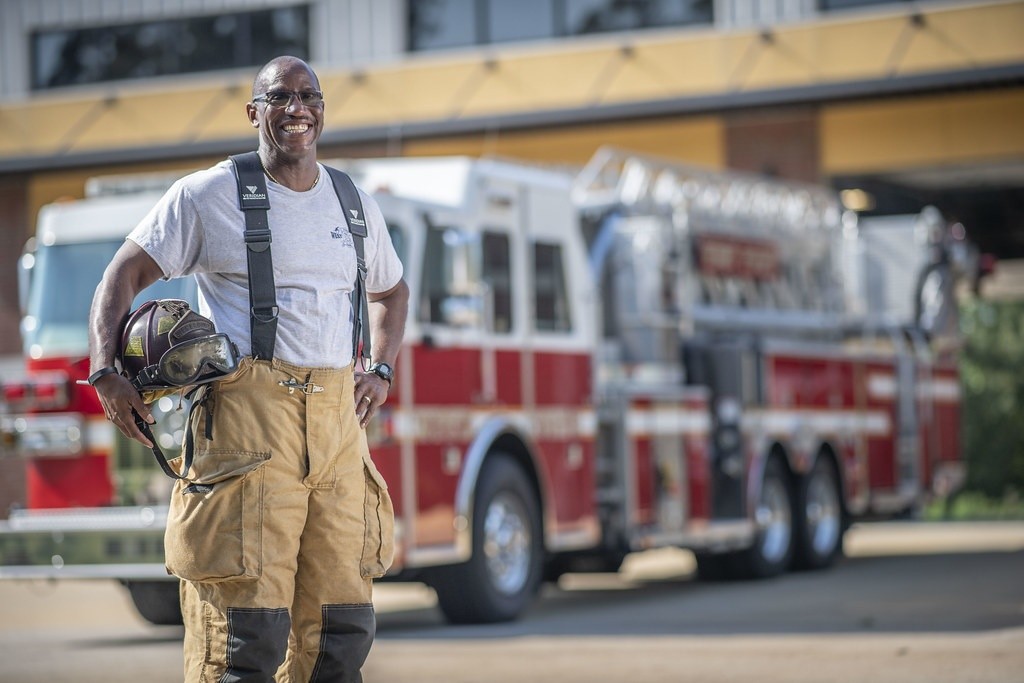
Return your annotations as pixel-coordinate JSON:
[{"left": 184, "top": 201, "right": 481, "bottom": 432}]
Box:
[{"left": 1, "top": 143, "right": 998, "bottom": 624}]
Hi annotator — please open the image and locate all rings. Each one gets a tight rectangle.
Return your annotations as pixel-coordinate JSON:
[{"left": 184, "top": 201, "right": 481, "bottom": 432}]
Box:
[{"left": 363, "top": 395, "right": 371, "bottom": 404}]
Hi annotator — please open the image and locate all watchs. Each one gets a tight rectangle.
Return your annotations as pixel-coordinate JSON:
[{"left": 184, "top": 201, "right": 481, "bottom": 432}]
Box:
[{"left": 368, "top": 363, "right": 394, "bottom": 388}]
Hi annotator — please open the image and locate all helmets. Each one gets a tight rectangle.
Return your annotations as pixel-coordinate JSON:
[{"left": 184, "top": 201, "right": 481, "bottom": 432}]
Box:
[{"left": 113, "top": 298, "right": 240, "bottom": 395}]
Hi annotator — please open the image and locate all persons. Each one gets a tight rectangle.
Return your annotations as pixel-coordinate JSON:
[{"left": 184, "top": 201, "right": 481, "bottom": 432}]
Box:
[{"left": 88, "top": 56, "right": 409, "bottom": 683}]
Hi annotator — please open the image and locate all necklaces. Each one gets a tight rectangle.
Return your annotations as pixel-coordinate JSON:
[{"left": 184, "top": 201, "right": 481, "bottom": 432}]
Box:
[{"left": 261, "top": 162, "right": 320, "bottom": 190}]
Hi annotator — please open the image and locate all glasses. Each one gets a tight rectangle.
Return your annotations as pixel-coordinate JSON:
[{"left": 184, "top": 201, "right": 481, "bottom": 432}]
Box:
[
  {"left": 252, "top": 90, "right": 324, "bottom": 107},
  {"left": 129, "top": 333, "right": 236, "bottom": 393}
]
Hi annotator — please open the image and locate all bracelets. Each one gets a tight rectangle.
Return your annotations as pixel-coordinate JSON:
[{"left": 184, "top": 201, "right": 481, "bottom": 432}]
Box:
[{"left": 88, "top": 367, "right": 118, "bottom": 386}]
